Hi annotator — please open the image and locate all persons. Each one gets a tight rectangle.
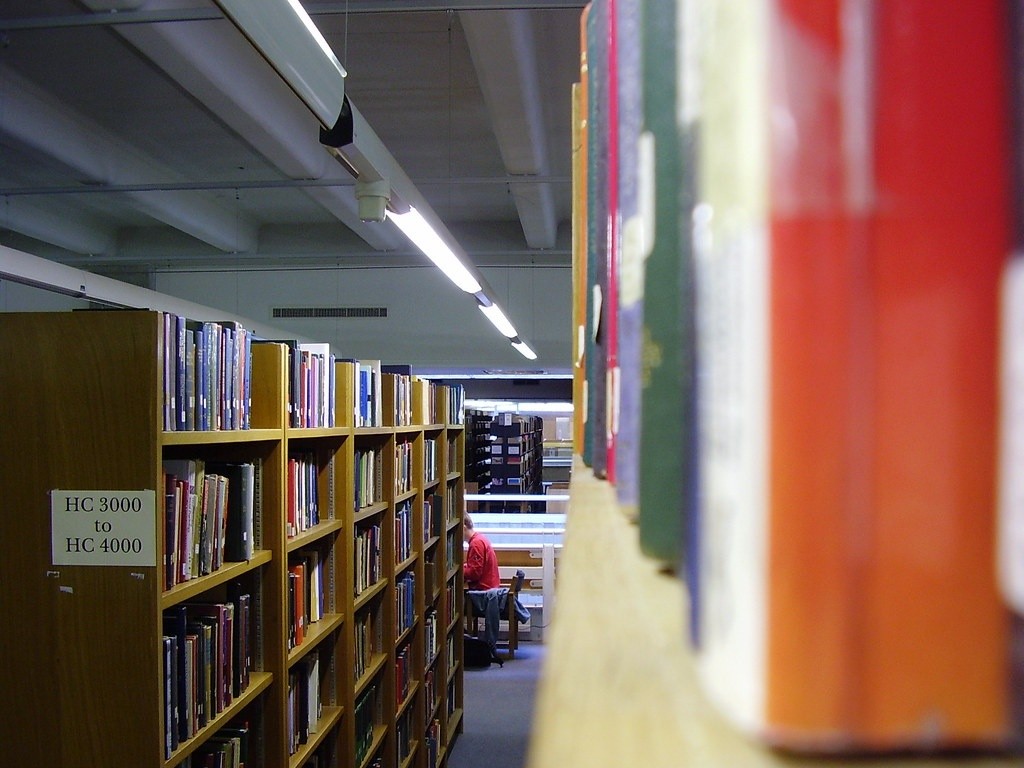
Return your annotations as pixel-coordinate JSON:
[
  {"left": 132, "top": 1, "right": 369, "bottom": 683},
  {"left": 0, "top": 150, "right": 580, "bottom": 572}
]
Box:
[{"left": 464, "top": 511, "right": 500, "bottom": 638}]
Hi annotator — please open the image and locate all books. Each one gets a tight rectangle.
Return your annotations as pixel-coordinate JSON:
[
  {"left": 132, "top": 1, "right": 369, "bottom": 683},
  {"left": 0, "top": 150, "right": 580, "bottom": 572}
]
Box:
[
  {"left": 577, "top": 4, "right": 1015, "bottom": 752},
  {"left": 159, "top": 313, "right": 463, "bottom": 768},
  {"left": 467, "top": 409, "right": 544, "bottom": 513}
]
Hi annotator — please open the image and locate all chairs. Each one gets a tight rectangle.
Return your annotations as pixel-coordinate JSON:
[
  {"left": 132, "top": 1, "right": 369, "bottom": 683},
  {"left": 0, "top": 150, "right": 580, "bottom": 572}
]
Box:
[{"left": 465, "top": 569, "right": 526, "bottom": 660}]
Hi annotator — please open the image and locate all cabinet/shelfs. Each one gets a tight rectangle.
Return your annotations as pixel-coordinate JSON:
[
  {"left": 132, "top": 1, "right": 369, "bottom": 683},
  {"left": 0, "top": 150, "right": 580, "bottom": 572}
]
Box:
[
  {"left": 465, "top": 407, "right": 545, "bottom": 514},
  {"left": 0, "top": 308, "right": 464, "bottom": 768}
]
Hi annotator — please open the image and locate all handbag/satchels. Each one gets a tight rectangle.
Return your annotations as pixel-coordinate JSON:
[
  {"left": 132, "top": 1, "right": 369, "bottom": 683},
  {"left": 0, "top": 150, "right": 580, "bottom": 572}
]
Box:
[{"left": 464, "top": 634, "right": 503, "bottom": 669}]
